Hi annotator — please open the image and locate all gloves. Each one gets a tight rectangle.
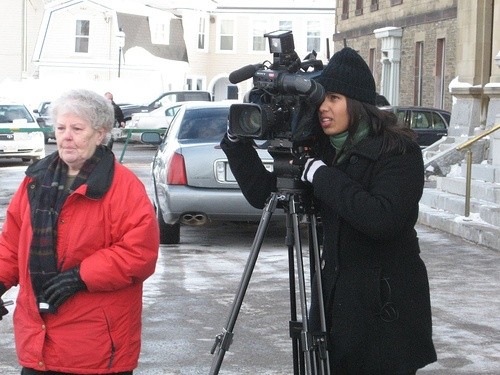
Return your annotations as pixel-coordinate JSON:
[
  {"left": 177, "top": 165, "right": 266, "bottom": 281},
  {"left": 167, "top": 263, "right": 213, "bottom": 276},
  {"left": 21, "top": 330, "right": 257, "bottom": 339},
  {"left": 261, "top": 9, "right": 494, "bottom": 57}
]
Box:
[
  {"left": 42, "top": 265, "right": 87, "bottom": 308},
  {"left": 226, "top": 115, "right": 240, "bottom": 143},
  {"left": 299, "top": 155, "right": 327, "bottom": 184},
  {"left": 0, "top": 280, "right": 9, "bottom": 321}
]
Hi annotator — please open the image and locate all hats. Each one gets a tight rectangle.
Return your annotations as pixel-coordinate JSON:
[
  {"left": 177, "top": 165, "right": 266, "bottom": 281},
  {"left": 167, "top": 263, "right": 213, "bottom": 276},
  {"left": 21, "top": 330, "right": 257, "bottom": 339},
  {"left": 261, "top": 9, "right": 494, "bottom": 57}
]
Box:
[{"left": 317, "top": 47, "right": 377, "bottom": 105}]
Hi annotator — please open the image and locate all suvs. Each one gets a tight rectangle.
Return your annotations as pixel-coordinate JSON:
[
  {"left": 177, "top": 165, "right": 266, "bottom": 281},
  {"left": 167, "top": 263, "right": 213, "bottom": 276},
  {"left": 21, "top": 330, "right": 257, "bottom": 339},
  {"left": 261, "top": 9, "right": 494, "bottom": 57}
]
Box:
[{"left": 120, "top": 90, "right": 211, "bottom": 123}]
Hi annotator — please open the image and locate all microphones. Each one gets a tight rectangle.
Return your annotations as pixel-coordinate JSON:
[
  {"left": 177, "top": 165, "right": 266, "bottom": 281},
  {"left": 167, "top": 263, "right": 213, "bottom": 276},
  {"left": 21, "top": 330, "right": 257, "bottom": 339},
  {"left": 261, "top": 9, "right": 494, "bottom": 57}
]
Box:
[{"left": 229, "top": 63, "right": 267, "bottom": 84}]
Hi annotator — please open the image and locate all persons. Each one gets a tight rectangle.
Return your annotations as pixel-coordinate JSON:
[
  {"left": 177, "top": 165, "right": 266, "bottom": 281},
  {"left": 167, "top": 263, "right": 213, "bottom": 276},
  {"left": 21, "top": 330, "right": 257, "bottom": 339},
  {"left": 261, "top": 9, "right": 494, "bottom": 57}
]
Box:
[
  {"left": 219, "top": 46, "right": 438, "bottom": 375},
  {"left": 104, "top": 92, "right": 126, "bottom": 151},
  {"left": 0, "top": 89, "right": 159, "bottom": 375}
]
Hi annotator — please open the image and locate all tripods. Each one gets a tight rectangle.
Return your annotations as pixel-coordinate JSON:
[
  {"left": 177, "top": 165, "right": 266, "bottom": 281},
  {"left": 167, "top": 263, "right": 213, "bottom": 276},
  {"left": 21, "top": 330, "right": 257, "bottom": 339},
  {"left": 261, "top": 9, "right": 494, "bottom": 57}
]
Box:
[{"left": 210, "top": 144, "right": 331, "bottom": 374}]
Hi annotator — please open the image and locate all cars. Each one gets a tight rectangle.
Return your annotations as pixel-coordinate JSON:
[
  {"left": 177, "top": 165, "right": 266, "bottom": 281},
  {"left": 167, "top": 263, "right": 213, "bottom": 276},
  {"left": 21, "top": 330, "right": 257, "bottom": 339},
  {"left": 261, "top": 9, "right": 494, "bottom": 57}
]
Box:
[
  {"left": 140, "top": 101, "right": 293, "bottom": 244},
  {"left": 0, "top": 104, "right": 46, "bottom": 161},
  {"left": 126, "top": 102, "right": 184, "bottom": 145},
  {"left": 380, "top": 106, "right": 452, "bottom": 146},
  {"left": 33, "top": 102, "right": 59, "bottom": 143}
]
